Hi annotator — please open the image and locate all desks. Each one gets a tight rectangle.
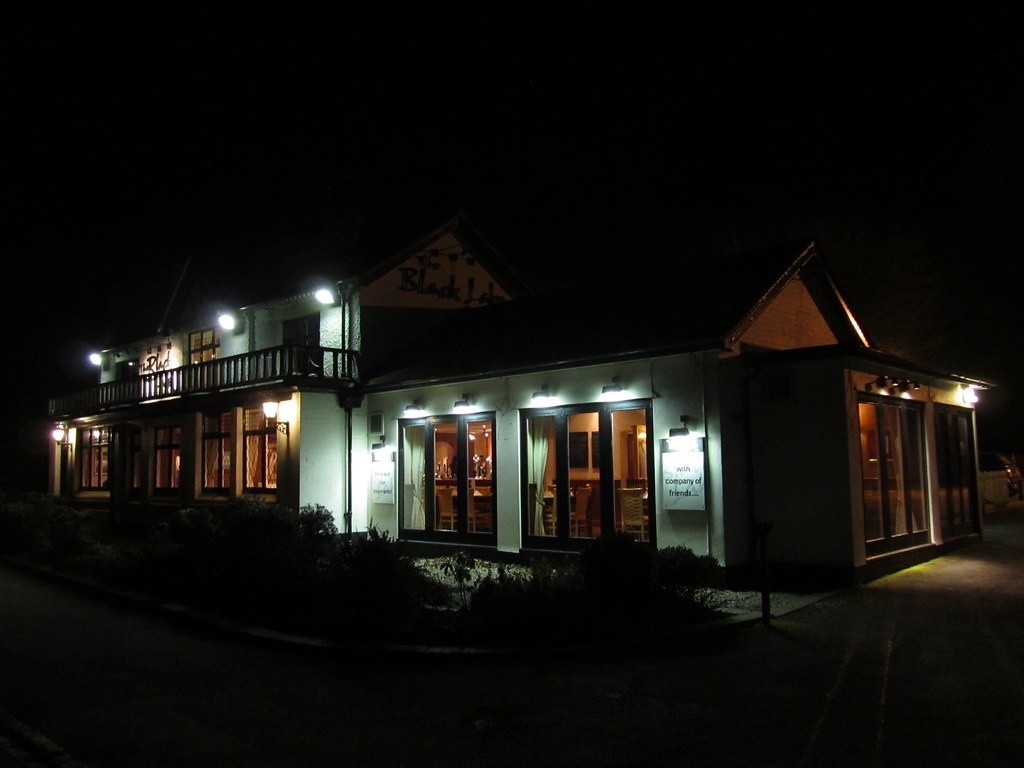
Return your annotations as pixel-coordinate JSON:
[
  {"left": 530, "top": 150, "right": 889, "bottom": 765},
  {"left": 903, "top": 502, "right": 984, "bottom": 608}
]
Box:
[
  {"left": 543, "top": 495, "right": 576, "bottom": 505},
  {"left": 452, "top": 495, "right": 491, "bottom": 504},
  {"left": 435, "top": 477, "right": 491, "bottom": 486}
]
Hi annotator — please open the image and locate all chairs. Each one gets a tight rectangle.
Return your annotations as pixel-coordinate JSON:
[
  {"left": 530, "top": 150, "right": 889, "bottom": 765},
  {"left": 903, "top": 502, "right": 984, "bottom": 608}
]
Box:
[
  {"left": 436, "top": 488, "right": 458, "bottom": 531},
  {"left": 543, "top": 488, "right": 558, "bottom": 535},
  {"left": 570, "top": 488, "right": 592, "bottom": 537}
]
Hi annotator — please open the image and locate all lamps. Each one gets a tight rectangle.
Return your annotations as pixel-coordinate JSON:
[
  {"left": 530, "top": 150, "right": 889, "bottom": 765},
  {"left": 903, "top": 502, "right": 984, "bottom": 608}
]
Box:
[
  {"left": 405, "top": 400, "right": 420, "bottom": 411},
  {"left": 867, "top": 376, "right": 898, "bottom": 389},
  {"left": 372, "top": 435, "right": 388, "bottom": 451},
  {"left": 51, "top": 425, "right": 72, "bottom": 452},
  {"left": 890, "top": 379, "right": 921, "bottom": 390},
  {"left": 454, "top": 393, "right": 472, "bottom": 407},
  {"left": 669, "top": 415, "right": 690, "bottom": 436},
  {"left": 638, "top": 432, "right": 647, "bottom": 443},
  {"left": 601, "top": 376, "right": 622, "bottom": 392},
  {"left": 263, "top": 402, "right": 288, "bottom": 435},
  {"left": 147, "top": 338, "right": 171, "bottom": 354},
  {"left": 532, "top": 384, "right": 549, "bottom": 400}
]
